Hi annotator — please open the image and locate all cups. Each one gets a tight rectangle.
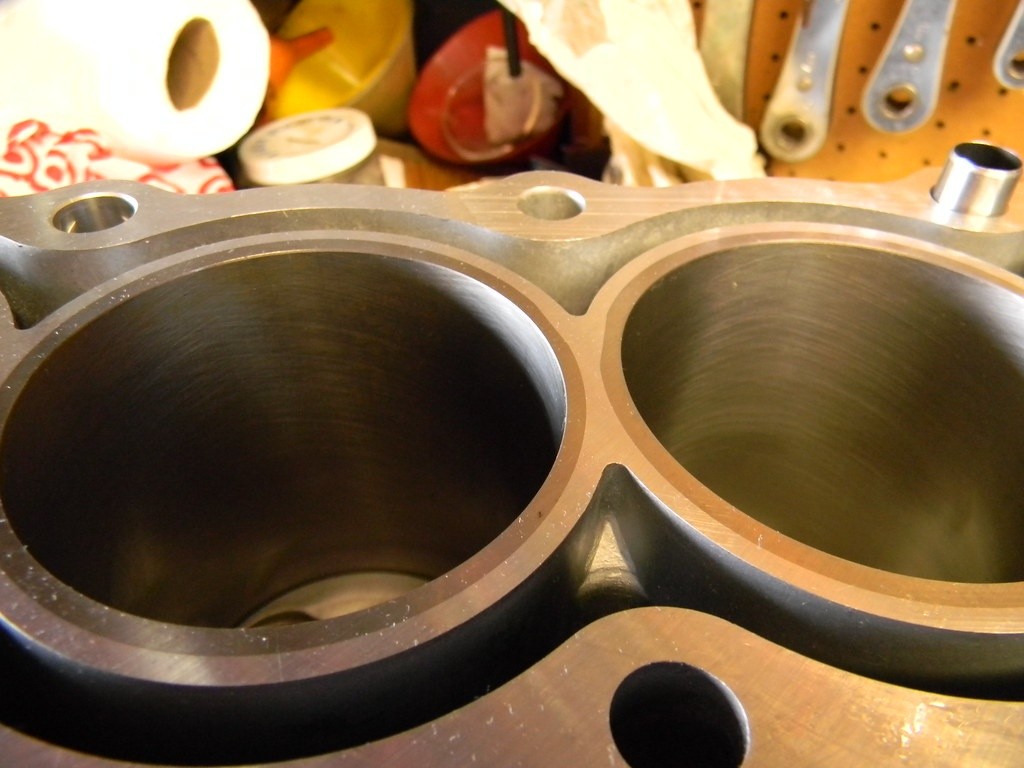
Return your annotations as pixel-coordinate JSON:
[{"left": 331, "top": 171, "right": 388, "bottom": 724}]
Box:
[{"left": 239, "top": 107, "right": 387, "bottom": 188}]
